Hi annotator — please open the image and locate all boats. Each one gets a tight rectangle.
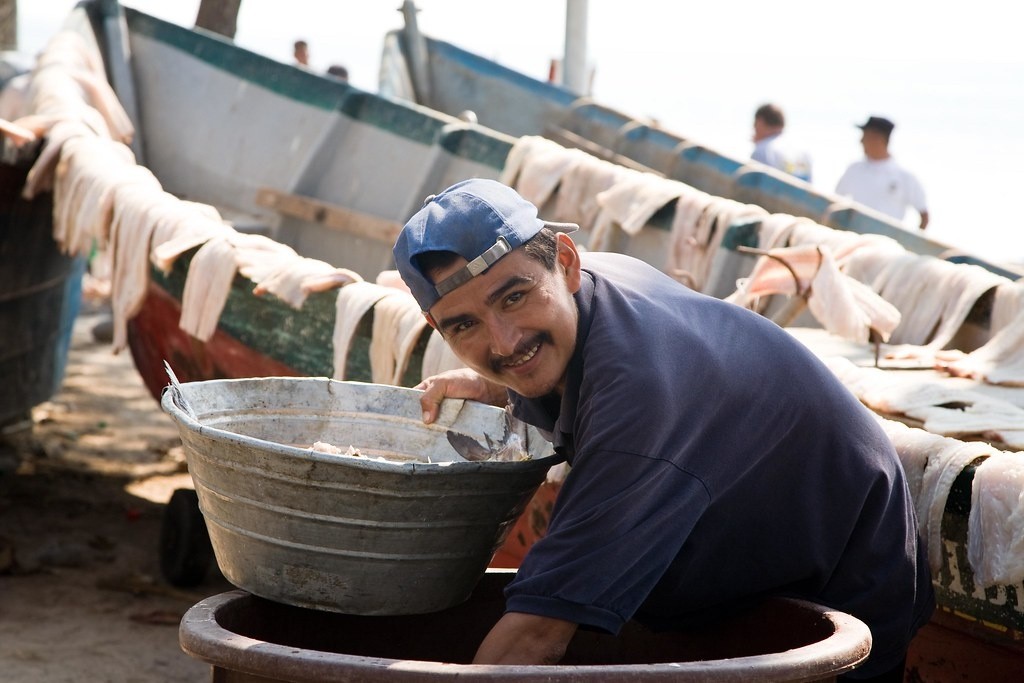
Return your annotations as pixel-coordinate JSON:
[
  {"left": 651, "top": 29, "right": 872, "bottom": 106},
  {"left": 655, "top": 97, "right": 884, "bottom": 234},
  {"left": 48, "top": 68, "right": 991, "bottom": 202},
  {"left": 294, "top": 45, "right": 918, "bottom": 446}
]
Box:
[
  {"left": 45, "top": 0, "right": 1024, "bottom": 683},
  {"left": 381, "top": 26, "right": 1023, "bottom": 286},
  {"left": 1, "top": 37, "right": 85, "bottom": 430}
]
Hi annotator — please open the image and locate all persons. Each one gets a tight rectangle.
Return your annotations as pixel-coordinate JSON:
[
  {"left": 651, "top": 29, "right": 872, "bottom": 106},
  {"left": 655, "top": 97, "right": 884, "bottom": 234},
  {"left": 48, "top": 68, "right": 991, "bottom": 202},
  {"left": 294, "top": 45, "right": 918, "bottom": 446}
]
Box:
[
  {"left": 293, "top": 40, "right": 348, "bottom": 81},
  {"left": 395, "top": 104, "right": 929, "bottom": 683}
]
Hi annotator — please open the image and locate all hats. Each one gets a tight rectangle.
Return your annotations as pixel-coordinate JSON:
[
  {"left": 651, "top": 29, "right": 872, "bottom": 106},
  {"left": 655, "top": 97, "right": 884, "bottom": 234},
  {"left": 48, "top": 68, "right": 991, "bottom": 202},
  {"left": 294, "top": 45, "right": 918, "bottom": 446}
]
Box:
[
  {"left": 858, "top": 116, "right": 894, "bottom": 136},
  {"left": 392, "top": 179, "right": 580, "bottom": 312}
]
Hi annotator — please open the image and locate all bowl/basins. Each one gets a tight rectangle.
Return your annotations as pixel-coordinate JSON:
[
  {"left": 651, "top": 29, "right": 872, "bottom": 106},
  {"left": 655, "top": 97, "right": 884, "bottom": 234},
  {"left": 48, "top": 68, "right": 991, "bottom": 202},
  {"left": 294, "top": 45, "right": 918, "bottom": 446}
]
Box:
[{"left": 161, "top": 377, "right": 562, "bottom": 619}]
